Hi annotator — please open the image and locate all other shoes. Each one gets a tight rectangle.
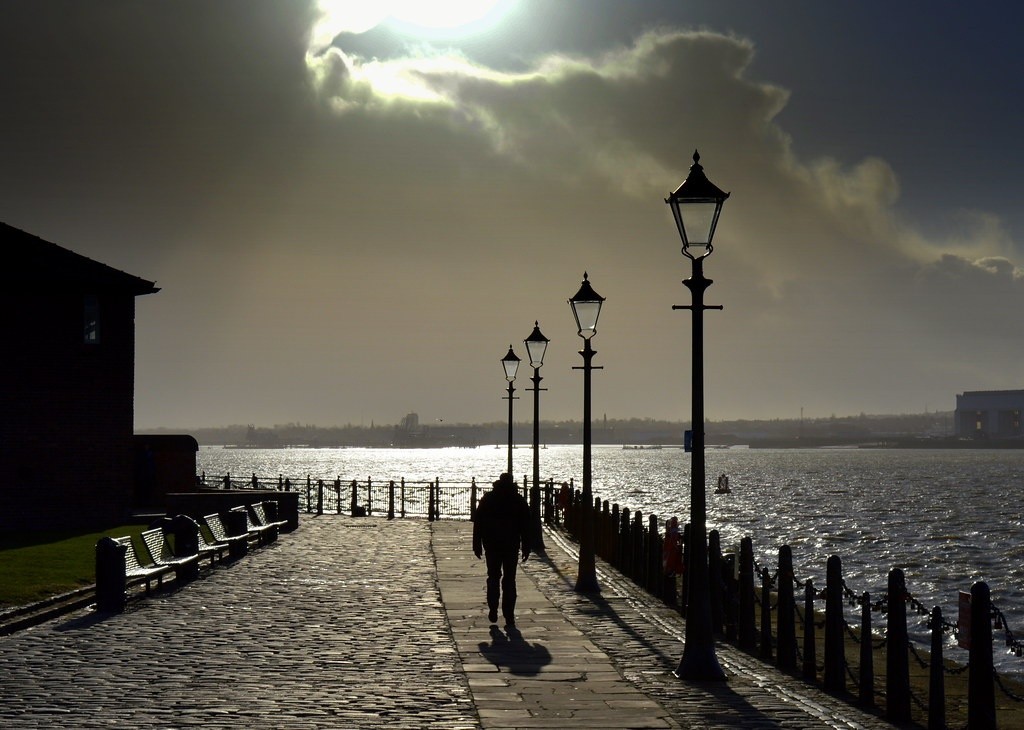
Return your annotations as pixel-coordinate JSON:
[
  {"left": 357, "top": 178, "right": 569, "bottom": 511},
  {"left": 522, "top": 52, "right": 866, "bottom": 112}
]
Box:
[
  {"left": 506, "top": 617, "right": 515, "bottom": 626},
  {"left": 488, "top": 601, "right": 499, "bottom": 622}
]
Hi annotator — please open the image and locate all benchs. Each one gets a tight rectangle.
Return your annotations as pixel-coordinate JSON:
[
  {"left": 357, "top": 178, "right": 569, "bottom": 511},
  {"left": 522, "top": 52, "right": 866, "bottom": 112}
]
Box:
[
  {"left": 201, "top": 513, "right": 251, "bottom": 563},
  {"left": 249, "top": 502, "right": 288, "bottom": 539},
  {"left": 192, "top": 520, "right": 229, "bottom": 570},
  {"left": 230, "top": 506, "right": 275, "bottom": 548},
  {"left": 115, "top": 536, "right": 169, "bottom": 596},
  {"left": 142, "top": 526, "right": 199, "bottom": 588}
]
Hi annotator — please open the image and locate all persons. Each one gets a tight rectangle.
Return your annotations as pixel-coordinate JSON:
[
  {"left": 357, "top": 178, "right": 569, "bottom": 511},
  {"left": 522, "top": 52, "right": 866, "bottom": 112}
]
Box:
[
  {"left": 559, "top": 483, "right": 569, "bottom": 508},
  {"left": 472, "top": 473, "right": 532, "bottom": 625}
]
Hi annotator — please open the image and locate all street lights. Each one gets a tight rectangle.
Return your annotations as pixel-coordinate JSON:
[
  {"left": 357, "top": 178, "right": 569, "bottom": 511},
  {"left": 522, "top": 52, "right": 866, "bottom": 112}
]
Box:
[
  {"left": 497, "top": 344, "right": 525, "bottom": 475},
  {"left": 521, "top": 320, "right": 551, "bottom": 551},
  {"left": 663, "top": 147, "right": 729, "bottom": 683},
  {"left": 564, "top": 268, "right": 602, "bottom": 594}
]
